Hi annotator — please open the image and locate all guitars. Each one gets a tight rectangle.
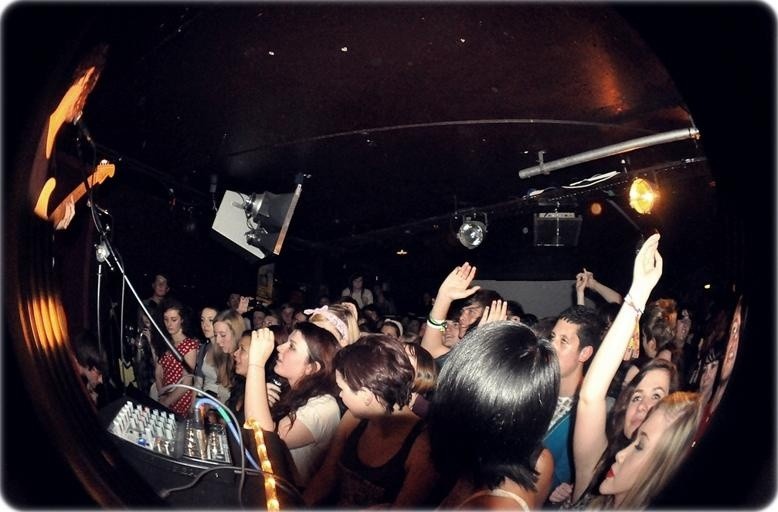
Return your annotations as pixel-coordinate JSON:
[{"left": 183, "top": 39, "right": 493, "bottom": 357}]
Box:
[{"left": 48, "top": 163, "right": 116, "bottom": 227}]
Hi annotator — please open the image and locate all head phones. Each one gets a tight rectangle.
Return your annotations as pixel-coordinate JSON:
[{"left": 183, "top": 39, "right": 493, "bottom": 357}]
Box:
[{"left": 94, "top": 233, "right": 117, "bottom": 262}]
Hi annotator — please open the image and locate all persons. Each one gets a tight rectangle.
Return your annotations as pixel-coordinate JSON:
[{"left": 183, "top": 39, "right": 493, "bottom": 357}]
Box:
[
  {"left": 29, "top": 65, "right": 115, "bottom": 230},
  {"left": 72, "top": 234, "right": 742, "bottom": 511}
]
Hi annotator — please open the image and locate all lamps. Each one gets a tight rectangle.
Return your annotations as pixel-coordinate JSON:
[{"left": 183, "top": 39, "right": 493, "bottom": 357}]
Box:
[
  {"left": 628, "top": 177, "right": 669, "bottom": 216},
  {"left": 209, "top": 180, "right": 305, "bottom": 259},
  {"left": 453, "top": 210, "right": 489, "bottom": 251}
]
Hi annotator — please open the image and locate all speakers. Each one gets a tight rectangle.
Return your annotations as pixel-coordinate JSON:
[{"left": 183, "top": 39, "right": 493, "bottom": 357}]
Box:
[{"left": 534, "top": 214, "right": 583, "bottom": 248}]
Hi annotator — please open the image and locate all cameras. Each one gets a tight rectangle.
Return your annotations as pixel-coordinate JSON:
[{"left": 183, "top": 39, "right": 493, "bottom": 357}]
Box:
[{"left": 248, "top": 299, "right": 263, "bottom": 307}]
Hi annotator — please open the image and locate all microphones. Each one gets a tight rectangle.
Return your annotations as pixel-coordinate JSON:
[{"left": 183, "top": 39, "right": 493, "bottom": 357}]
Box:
[
  {"left": 73, "top": 110, "right": 94, "bottom": 142},
  {"left": 103, "top": 256, "right": 115, "bottom": 272}
]
